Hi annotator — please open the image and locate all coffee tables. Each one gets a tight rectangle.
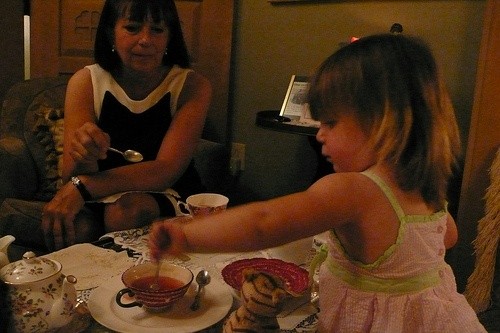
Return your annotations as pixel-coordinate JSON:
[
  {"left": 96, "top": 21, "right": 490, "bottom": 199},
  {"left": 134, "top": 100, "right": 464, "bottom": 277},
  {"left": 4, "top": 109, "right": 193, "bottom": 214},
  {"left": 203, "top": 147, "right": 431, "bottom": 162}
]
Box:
[{"left": 41, "top": 216, "right": 330, "bottom": 333}]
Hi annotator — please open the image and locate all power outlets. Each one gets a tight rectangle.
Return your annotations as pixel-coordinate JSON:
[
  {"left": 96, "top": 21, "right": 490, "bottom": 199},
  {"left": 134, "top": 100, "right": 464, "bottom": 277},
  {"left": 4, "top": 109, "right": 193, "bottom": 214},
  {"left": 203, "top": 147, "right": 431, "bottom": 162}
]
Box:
[{"left": 230, "top": 141, "right": 245, "bottom": 170}]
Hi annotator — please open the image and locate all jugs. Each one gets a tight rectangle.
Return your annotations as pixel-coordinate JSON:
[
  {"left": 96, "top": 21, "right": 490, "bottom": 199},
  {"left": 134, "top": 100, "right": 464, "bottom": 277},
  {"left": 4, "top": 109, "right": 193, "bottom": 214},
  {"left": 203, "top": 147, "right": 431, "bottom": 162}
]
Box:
[{"left": 0, "top": 235, "right": 16, "bottom": 266}]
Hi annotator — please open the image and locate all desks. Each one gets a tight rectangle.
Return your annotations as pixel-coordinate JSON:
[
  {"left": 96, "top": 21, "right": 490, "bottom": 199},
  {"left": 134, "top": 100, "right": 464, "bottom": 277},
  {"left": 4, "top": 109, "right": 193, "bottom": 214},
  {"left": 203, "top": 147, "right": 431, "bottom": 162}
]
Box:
[{"left": 254, "top": 110, "right": 335, "bottom": 182}]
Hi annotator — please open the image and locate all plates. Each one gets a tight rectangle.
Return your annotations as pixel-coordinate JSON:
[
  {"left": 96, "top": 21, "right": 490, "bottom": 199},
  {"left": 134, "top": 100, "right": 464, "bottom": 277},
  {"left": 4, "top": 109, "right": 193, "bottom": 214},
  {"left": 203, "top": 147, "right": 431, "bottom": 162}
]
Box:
[{"left": 87, "top": 272, "right": 234, "bottom": 333}]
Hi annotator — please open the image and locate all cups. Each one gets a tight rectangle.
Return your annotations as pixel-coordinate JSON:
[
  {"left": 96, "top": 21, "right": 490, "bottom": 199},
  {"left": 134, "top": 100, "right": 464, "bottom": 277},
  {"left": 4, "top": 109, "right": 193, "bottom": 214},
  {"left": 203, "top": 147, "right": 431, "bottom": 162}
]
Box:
[
  {"left": 176, "top": 193, "right": 229, "bottom": 220},
  {"left": 115, "top": 261, "right": 194, "bottom": 312}
]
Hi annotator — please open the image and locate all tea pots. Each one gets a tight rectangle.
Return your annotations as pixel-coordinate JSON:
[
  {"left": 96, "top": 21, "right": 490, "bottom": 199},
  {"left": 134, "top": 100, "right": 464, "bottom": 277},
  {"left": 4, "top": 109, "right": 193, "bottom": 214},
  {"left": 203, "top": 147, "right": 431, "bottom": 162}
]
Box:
[{"left": 0, "top": 252, "right": 77, "bottom": 333}]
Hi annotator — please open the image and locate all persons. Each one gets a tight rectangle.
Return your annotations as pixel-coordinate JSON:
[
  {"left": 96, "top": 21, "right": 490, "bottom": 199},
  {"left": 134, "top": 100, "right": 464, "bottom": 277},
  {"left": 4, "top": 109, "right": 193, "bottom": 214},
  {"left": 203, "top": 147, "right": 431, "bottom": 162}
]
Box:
[
  {"left": 41, "top": 0, "right": 212, "bottom": 252},
  {"left": 147, "top": 31, "right": 489, "bottom": 333}
]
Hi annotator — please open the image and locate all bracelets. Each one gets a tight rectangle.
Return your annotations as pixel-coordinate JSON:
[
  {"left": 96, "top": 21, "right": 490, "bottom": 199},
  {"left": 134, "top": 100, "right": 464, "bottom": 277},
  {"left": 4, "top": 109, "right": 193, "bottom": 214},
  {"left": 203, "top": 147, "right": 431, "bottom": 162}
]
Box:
[{"left": 68, "top": 176, "right": 95, "bottom": 205}]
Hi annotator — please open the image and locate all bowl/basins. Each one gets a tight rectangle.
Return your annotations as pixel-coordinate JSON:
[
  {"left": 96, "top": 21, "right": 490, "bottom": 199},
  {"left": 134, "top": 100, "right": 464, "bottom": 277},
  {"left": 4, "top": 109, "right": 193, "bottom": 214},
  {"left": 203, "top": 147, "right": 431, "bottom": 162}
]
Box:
[{"left": 222, "top": 257, "right": 314, "bottom": 298}]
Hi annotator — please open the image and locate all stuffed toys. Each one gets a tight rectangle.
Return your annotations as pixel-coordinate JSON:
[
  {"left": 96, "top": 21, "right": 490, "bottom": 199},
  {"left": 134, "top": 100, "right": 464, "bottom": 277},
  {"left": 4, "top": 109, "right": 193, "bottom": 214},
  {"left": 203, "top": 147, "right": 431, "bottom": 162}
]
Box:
[{"left": 222, "top": 267, "right": 288, "bottom": 333}]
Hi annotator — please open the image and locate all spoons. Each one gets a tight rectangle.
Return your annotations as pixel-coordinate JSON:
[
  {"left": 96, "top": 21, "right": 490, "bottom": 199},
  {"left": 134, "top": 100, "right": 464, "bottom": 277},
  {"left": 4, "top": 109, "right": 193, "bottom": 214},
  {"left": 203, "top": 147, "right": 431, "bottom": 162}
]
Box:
[
  {"left": 190, "top": 270, "right": 211, "bottom": 312},
  {"left": 108, "top": 147, "right": 143, "bottom": 162}
]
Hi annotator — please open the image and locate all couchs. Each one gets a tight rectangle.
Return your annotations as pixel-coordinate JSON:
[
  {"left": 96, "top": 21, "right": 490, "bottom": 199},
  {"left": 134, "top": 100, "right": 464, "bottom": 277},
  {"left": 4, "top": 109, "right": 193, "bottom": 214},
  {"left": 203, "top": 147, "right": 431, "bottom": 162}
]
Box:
[{"left": 0, "top": 75, "right": 239, "bottom": 263}]
